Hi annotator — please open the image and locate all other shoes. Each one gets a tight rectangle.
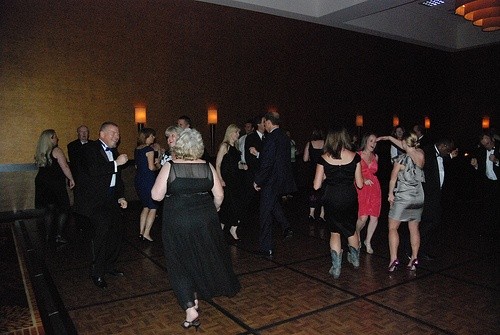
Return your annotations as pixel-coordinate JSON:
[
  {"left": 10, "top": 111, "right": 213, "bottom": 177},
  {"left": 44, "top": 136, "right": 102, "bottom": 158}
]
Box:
[
  {"left": 229, "top": 230, "right": 242, "bottom": 243},
  {"left": 265, "top": 249, "right": 273, "bottom": 256},
  {"left": 56, "top": 237, "right": 71, "bottom": 243},
  {"left": 281, "top": 227, "right": 294, "bottom": 242}
]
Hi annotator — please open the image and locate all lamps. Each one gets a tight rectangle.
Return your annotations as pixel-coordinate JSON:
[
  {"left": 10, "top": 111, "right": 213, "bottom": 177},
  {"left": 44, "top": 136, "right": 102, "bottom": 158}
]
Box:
[
  {"left": 392, "top": 115, "right": 400, "bottom": 128},
  {"left": 355, "top": 115, "right": 364, "bottom": 147},
  {"left": 207, "top": 109, "right": 218, "bottom": 154},
  {"left": 134, "top": 107, "right": 147, "bottom": 131},
  {"left": 455, "top": 0, "right": 500, "bottom": 32},
  {"left": 481, "top": 111, "right": 491, "bottom": 135}
]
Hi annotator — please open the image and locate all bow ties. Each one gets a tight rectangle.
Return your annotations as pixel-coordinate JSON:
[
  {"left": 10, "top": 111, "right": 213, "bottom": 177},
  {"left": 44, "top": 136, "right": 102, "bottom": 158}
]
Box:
[
  {"left": 435, "top": 150, "right": 444, "bottom": 158},
  {"left": 100, "top": 140, "right": 113, "bottom": 152}
]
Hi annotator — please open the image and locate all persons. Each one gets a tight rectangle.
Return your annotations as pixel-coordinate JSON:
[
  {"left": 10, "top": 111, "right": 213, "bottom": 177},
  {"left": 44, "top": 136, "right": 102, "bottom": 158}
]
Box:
[
  {"left": 385, "top": 126, "right": 426, "bottom": 196},
  {"left": 423, "top": 134, "right": 500, "bottom": 241},
  {"left": 351, "top": 133, "right": 382, "bottom": 254},
  {"left": 375, "top": 130, "right": 425, "bottom": 273},
  {"left": 152, "top": 129, "right": 240, "bottom": 329},
  {"left": 303, "top": 128, "right": 328, "bottom": 223},
  {"left": 67, "top": 125, "right": 95, "bottom": 193},
  {"left": 149, "top": 117, "right": 191, "bottom": 169},
  {"left": 314, "top": 126, "right": 364, "bottom": 279},
  {"left": 238, "top": 110, "right": 298, "bottom": 249},
  {"left": 135, "top": 127, "right": 160, "bottom": 244},
  {"left": 215, "top": 124, "right": 249, "bottom": 245},
  {"left": 75, "top": 122, "right": 130, "bottom": 290},
  {"left": 32, "top": 129, "right": 75, "bottom": 251}
]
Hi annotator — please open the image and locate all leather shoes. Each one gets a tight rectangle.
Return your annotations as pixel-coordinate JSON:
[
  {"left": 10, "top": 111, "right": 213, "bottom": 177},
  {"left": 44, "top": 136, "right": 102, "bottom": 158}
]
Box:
[
  {"left": 94, "top": 276, "right": 108, "bottom": 289},
  {"left": 109, "top": 269, "right": 127, "bottom": 278}
]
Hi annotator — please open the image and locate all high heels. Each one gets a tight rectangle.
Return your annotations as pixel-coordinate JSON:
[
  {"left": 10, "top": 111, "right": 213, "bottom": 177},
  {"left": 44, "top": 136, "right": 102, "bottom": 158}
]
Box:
[
  {"left": 183, "top": 317, "right": 201, "bottom": 331},
  {"left": 309, "top": 215, "right": 316, "bottom": 224},
  {"left": 139, "top": 234, "right": 154, "bottom": 243},
  {"left": 406, "top": 259, "right": 419, "bottom": 272},
  {"left": 318, "top": 217, "right": 328, "bottom": 225},
  {"left": 388, "top": 259, "right": 400, "bottom": 273}
]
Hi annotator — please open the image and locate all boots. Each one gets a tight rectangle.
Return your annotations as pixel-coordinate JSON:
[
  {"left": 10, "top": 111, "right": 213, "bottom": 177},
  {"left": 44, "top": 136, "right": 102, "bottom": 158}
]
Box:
[
  {"left": 328, "top": 248, "right": 344, "bottom": 278},
  {"left": 347, "top": 245, "right": 360, "bottom": 270}
]
